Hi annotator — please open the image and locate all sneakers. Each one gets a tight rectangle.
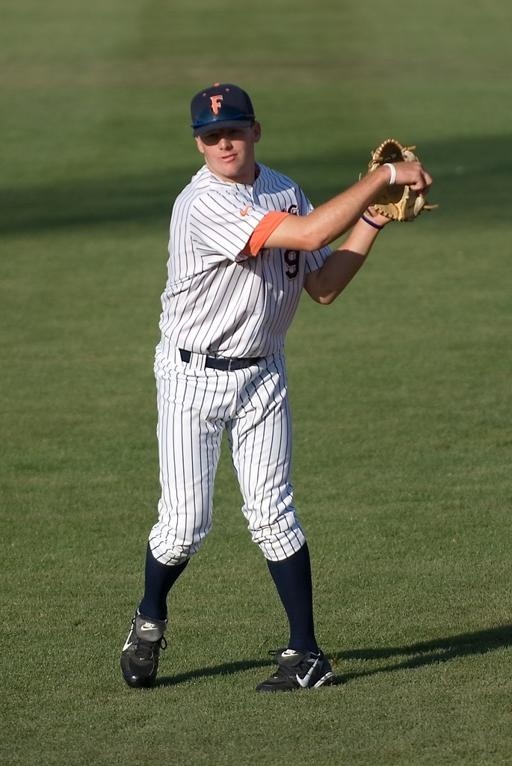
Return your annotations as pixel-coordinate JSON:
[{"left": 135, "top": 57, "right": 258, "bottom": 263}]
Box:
[
  {"left": 121, "top": 608, "right": 167, "bottom": 687},
  {"left": 256, "top": 648, "right": 335, "bottom": 689}
]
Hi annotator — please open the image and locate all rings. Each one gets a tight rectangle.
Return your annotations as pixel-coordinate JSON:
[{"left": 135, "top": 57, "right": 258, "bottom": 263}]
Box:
[{"left": 424, "top": 171, "right": 429, "bottom": 179}]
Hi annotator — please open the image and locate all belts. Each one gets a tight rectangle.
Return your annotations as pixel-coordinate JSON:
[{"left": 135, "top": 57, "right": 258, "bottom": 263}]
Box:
[{"left": 179, "top": 349, "right": 262, "bottom": 371}]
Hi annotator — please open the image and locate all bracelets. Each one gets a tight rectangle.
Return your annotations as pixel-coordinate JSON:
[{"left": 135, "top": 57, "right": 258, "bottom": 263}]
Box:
[
  {"left": 360, "top": 212, "right": 386, "bottom": 229},
  {"left": 382, "top": 161, "right": 396, "bottom": 187}
]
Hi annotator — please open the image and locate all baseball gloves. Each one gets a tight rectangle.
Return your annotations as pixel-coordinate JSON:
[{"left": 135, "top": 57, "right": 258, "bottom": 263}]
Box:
[{"left": 368, "top": 139, "right": 439, "bottom": 222}]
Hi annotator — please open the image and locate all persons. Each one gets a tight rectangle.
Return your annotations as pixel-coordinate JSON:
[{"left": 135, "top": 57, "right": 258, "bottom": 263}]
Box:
[{"left": 120, "top": 82, "right": 432, "bottom": 693}]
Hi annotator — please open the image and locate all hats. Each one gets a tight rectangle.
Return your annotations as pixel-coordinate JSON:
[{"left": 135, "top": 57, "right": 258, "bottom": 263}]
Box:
[{"left": 191, "top": 82, "right": 256, "bottom": 136}]
